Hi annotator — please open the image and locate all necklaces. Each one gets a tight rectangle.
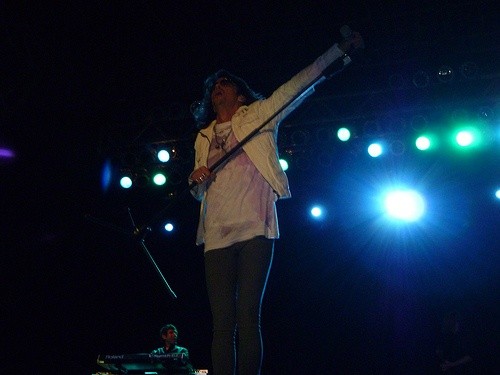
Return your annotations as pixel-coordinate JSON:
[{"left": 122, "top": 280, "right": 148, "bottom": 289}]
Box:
[{"left": 211, "top": 121, "right": 232, "bottom": 150}]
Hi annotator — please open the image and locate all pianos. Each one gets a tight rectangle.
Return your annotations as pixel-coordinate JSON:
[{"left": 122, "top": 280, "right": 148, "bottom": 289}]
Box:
[{"left": 97, "top": 352, "right": 190, "bottom": 371}]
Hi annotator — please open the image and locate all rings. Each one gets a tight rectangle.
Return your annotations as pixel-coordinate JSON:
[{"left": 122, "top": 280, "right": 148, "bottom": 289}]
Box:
[
  {"left": 195, "top": 178, "right": 200, "bottom": 183},
  {"left": 202, "top": 173, "right": 206, "bottom": 178},
  {"left": 199, "top": 176, "right": 204, "bottom": 181}
]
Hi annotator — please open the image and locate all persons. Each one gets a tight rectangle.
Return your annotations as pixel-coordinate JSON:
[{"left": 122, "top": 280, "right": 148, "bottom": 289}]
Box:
[
  {"left": 150, "top": 323, "right": 188, "bottom": 357},
  {"left": 188, "top": 28, "right": 363, "bottom": 375}
]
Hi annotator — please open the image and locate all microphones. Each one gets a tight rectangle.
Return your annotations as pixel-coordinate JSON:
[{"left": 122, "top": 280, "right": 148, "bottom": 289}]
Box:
[{"left": 339, "top": 24, "right": 360, "bottom": 57}]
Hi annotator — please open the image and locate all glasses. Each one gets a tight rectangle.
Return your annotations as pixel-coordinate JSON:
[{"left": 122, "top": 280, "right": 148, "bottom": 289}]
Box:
[{"left": 210, "top": 78, "right": 233, "bottom": 89}]
